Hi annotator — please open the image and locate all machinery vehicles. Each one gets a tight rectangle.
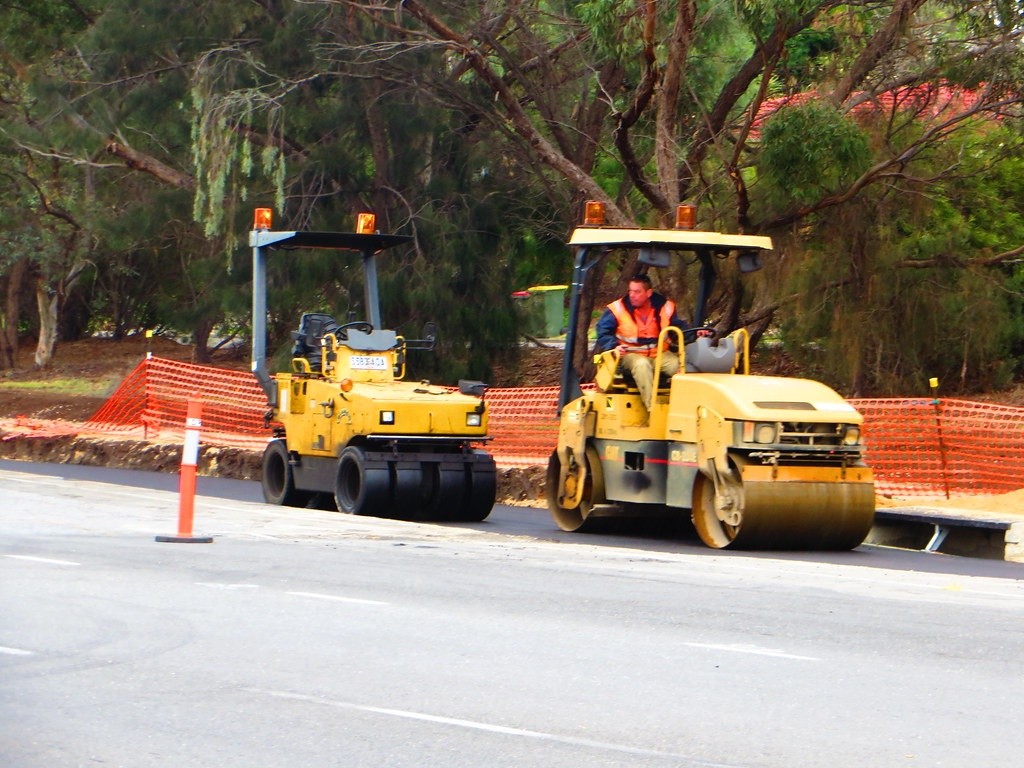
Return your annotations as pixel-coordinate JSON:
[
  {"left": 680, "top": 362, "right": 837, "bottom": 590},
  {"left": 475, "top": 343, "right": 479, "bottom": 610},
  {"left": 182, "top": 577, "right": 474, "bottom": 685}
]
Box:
[
  {"left": 546, "top": 202, "right": 875, "bottom": 551},
  {"left": 249, "top": 207, "right": 501, "bottom": 522}
]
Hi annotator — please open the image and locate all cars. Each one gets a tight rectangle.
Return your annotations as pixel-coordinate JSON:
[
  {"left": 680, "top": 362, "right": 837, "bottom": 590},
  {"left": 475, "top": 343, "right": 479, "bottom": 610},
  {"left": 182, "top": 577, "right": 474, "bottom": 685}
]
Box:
[
  {"left": 757, "top": 329, "right": 824, "bottom": 344},
  {"left": 513, "top": 284, "right": 603, "bottom": 341}
]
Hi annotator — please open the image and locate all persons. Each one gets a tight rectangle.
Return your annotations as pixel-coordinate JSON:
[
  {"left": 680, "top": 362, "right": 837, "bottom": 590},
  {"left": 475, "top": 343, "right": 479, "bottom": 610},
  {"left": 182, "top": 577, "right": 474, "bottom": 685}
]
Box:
[{"left": 595, "top": 274, "right": 712, "bottom": 427}]
